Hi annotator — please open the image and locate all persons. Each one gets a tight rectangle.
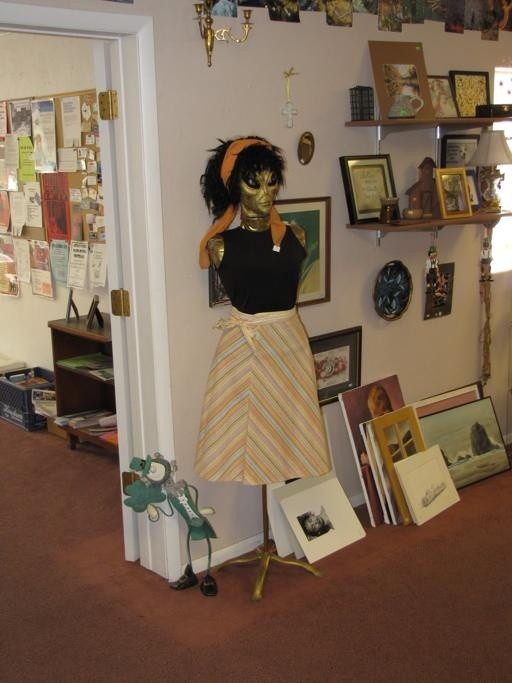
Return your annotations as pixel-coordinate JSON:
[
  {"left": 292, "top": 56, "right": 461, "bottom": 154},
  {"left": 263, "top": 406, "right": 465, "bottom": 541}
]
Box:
[
  {"left": 194, "top": 134, "right": 332, "bottom": 486},
  {"left": 296, "top": 505, "right": 334, "bottom": 537},
  {"left": 479, "top": 241, "right": 494, "bottom": 283},
  {"left": 425, "top": 246, "right": 440, "bottom": 293}
]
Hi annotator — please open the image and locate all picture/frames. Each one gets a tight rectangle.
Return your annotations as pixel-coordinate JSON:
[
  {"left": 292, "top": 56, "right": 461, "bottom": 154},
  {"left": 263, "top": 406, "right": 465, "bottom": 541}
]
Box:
[
  {"left": 466, "top": 170, "right": 482, "bottom": 210},
  {"left": 367, "top": 41, "right": 492, "bottom": 117},
  {"left": 435, "top": 168, "right": 472, "bottom": 219},
  {"left": 423, "top": 263, "right": 454, "bottom": 321},
  {"left": 273, "top": 197, "right": 332, "bottom": 306},
  {"left": 209, "top": 256, "right": 232, "bottom": 306},
  {"left": 66, "top": 288, "right": 78, "bottom": 322},
  {"left": 340, "top": 155, "right": 400, "bottom": 224},
  {"left": 86, "top": 295, "right": 103, "bottom": 329}
]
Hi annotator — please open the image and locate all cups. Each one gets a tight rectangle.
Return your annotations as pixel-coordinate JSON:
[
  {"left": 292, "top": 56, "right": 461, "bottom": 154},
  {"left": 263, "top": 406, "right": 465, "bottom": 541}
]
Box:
[{"left": 378, "top": 197, "right": 402, "bottom": 223}]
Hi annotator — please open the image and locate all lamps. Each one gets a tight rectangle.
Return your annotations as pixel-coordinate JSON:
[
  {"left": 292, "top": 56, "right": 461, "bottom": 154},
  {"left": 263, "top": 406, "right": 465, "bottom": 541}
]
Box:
[
  {"left": 193, "top": 0, "right": 254, "bottom": 68},
  {"left": 465, "top": 131, "right": 512, "bottom": 213}
]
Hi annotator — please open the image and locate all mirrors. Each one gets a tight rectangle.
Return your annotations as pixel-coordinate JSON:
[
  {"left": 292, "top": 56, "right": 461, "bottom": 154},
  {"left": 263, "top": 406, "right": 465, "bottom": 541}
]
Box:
[{"left": 299, "top": 132, "right": 315, "bottom": 165}]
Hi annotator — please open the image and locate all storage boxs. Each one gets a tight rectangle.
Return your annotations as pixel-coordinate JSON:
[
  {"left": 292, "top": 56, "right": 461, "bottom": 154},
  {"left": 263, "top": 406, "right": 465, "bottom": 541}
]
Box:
[{"left": 1, "top": 366, "right": 54, "bottom": 434}]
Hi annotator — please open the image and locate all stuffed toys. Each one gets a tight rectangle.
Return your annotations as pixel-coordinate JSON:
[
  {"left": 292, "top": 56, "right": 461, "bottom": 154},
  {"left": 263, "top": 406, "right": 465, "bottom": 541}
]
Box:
[{"left": 123, "top": 454, "right": 218, "bottom": 597}]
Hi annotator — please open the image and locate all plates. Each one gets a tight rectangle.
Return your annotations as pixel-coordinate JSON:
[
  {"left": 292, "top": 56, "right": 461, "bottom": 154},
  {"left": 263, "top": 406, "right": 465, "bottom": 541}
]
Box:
[{"left": 373, "top": 261, "right": 413, "bottom": 321}]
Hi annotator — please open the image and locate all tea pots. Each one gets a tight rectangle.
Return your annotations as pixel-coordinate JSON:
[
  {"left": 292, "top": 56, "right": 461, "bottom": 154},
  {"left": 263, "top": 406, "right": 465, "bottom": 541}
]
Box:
[{"left": 385, "top": 93, "right": 426, "bottom": 118}]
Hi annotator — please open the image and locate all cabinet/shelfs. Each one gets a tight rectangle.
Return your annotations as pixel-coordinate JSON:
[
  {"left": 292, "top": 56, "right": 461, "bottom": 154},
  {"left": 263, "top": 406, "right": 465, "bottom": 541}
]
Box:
[{"left": 47, "top": 311, "right": 117, "bottom": 451}]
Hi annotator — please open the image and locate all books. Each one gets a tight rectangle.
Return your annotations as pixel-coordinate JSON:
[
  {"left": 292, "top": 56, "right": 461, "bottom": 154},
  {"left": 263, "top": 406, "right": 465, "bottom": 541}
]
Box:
[{"left": 30, "top": 389, "right": 118, "bottom": 448}]
